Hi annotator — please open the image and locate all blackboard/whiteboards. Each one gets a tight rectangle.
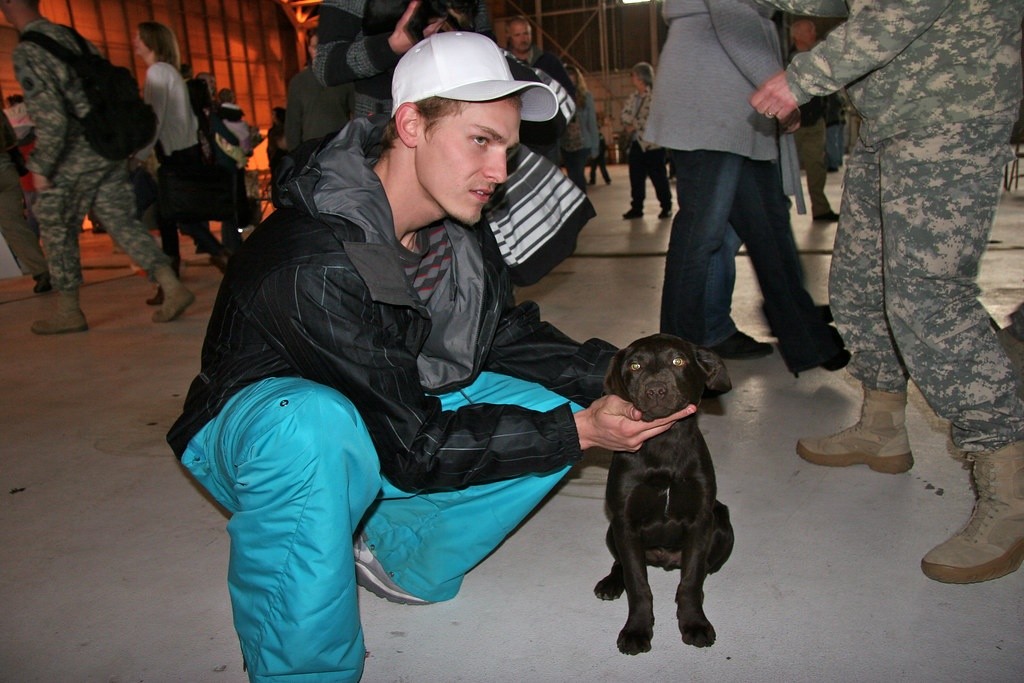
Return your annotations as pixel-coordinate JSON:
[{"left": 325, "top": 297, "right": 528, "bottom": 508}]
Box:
[{"left": 1010, "top": 99, "right": 1024, "bottom": 144}]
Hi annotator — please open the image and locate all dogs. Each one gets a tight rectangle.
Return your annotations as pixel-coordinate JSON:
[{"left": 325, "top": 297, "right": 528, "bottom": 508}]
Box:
[{"left": 593, "top": 334, "right": 736, "bottom": 654}]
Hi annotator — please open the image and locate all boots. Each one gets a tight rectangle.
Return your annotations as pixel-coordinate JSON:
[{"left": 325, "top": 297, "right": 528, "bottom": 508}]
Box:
[
  {"left": 921, "top": 438, "right": 1024, "bottom": 583},
  {"left": 31, "top": 289, "right": 88, "bottom": 334},
  {"left": 151, "top": 266, "right": 196, "bottom": 322},
  {"left": 796, "top": 384, "right": 914, "bottom": 474}
]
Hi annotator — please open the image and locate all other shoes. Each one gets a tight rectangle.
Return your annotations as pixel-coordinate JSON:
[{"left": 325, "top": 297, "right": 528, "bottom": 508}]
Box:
[
  {"left": 146, "top": 286, "right": 165, "bottom": 305},
  {"left": 354, "top": 533, "right": 435, "bottom": 604},
  {"left": 815, "top": 307, "right": 835, "bottom": 323},
  {"left": 623, "top": 210, "right": 643, "bottom": 217},
  {"left": 813, "top": 210, "right": 840, "bottom": 220},
  {"left": 709, "top": 332, "right": 773, "bottom": 360},
  {"left": 659, "top": 210, "right": 672, "bottom": 217},
  {"left": 827, "top": 166, "right": 838, "bottom": 171},
  {"left": 34, "top": 281, "right": 51, "bottom": 292}
]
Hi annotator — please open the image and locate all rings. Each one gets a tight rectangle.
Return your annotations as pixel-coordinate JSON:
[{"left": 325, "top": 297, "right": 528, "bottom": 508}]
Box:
[{"left": 767, "top": 111, "right": 775, "bottom": 116}]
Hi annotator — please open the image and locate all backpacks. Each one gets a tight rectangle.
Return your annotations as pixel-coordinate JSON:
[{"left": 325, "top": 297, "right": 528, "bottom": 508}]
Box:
[{"left": 18, "top": 24, "right": 159, "bottom": 160}]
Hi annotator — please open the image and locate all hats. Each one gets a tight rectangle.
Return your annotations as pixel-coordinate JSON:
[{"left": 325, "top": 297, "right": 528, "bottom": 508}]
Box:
[{"left": 392, "top": 31, "right": 558, "bottom": 121}]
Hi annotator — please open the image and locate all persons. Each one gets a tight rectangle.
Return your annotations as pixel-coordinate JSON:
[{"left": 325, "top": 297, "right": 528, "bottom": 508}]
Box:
[
  {"left": 136, "top": 23, "right": 254, "bottom": 305},
  {"left": 643, "top": 0, "right": 851, "bottom": 378},
  {"left": 621, "top": 62, "right": 672, "bottom": 220},
  {"left": 267, "top": 106, "right": 286, "bottom": 208},
  {"left": 784, "top": 19, "right": 840, "bottom": 222},
  {"left": 0, "top": 112, "right": 51, "bottom": 292},
  {"left": 285, "top": 27, "right": 354, "bottom": 175},
  {"left": 505, "top": 15, "right": 575, "bottom": 167},
  {"left": 748, "top": 0, "right": 1024, "bottom": 584},
  {"left": 166, "top": 31, "right": 697, "bottom": 683},
  {"left": 588, "top": 132, "right": 612, "bottom": 186},
  {"left": 565, "top": 65, "right": 599, "bottom": 195},
  {"left": 0, "top": 0, "right": 194, "bottom": 336},
  {"left": 312, "top": 0, "right": 498, "bottom": 120},
  {"left": 823, "top": 94, "right": 847, "bottom": 173}
]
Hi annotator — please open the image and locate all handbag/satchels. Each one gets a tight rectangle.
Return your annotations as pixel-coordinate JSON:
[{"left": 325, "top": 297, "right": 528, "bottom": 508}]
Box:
[
  {"left": 187, "top": 78, "right": 212, "bottom": 107},
  {"left": 618, "top": 130, "right": 633, "bottom": 151}
]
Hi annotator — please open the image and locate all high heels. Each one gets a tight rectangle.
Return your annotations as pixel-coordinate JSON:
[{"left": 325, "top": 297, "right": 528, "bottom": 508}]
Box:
[{"left": 795, "top": 349, "right": 851, "bottom": 378}]
